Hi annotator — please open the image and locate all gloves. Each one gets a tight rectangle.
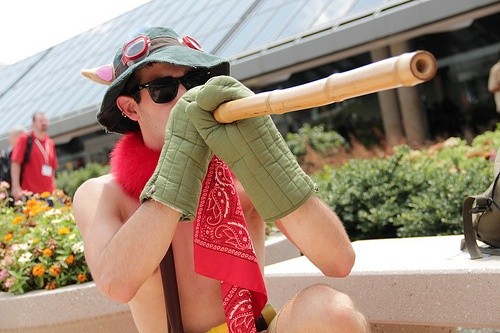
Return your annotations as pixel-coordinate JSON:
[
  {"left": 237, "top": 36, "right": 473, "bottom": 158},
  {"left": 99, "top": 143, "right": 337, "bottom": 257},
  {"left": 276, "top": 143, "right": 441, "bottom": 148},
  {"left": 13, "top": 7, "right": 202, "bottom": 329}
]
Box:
[
  {"left": 139, "top": 85, "right": 213, "bottom": 221},
  {"left": 185, "top": 76, "right": 314, "bottom": 222}
]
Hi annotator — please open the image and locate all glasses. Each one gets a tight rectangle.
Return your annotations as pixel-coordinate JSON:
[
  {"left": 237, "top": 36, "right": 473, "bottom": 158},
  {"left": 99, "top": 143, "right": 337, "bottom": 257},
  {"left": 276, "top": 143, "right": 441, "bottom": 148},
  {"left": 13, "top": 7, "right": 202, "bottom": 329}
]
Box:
[
  {"left": 126, "top": 69, "right": 211, "bottom": 103},
  {"left": 122, "top": 35, "right": 204, "bottom": 60}
]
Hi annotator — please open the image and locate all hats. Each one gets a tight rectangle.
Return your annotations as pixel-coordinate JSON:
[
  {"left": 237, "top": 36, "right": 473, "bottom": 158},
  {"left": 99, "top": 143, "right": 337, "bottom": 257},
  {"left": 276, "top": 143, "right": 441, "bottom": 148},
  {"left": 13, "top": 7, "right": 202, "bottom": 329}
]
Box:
[{"left": 82, "top": 27, "right": 231, "bottom": 134}]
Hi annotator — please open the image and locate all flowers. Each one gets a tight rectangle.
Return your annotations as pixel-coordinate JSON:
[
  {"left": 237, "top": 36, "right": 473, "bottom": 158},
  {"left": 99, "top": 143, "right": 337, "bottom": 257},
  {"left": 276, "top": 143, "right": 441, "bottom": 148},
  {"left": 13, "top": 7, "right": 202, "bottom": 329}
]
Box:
[{"left": 0, "top": 180, "right": 283, "bottom": 295}]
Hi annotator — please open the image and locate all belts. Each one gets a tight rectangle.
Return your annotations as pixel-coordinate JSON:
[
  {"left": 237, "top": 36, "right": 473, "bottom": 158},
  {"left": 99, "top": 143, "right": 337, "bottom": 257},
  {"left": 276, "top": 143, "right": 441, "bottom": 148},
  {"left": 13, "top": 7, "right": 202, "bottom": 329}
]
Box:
[{"left": 203, "top": 303, "right": 276, "bottom": 333}]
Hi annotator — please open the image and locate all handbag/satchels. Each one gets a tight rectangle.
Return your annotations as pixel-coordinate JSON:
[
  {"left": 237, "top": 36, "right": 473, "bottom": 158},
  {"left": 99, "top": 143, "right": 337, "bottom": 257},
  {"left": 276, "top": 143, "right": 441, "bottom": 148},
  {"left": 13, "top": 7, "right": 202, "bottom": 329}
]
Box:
[
  {"left": 462, "top": 170, "right": 500, "bottom": 249},
  {"left": 0, "top": 132, "right": 33, "bottom": 191}
]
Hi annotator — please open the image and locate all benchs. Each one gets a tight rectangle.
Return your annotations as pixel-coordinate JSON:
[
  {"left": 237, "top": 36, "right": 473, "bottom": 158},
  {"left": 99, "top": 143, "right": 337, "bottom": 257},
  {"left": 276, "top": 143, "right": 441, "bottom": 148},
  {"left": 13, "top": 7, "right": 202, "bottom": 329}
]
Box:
[{"left": 264, "top": 232, "right": 500, "bottom": 333}]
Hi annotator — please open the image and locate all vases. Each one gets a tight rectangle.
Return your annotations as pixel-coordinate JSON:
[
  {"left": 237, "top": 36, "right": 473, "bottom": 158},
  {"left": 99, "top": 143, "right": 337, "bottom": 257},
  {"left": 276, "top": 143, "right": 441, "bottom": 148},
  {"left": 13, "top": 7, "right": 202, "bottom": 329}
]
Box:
[{"left": 0, "top": 233, "right": 302, "bottom": 333}]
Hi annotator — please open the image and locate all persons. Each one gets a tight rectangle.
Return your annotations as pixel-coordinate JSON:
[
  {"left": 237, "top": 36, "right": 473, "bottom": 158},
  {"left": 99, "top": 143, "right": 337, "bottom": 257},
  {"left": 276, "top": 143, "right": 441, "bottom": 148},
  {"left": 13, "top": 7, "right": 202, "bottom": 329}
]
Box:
[
  {"left": 487, "top": 60, "right": 500, "bottom": 112},
  {"left": 10, "top": 113, "right": 59, "bottom": 201},
  {"left": 0, "top": 126, "right": 26, "bottom": 196},
  {"left": 72, "top": 27, "right": 371, "bottom": 333}
]
